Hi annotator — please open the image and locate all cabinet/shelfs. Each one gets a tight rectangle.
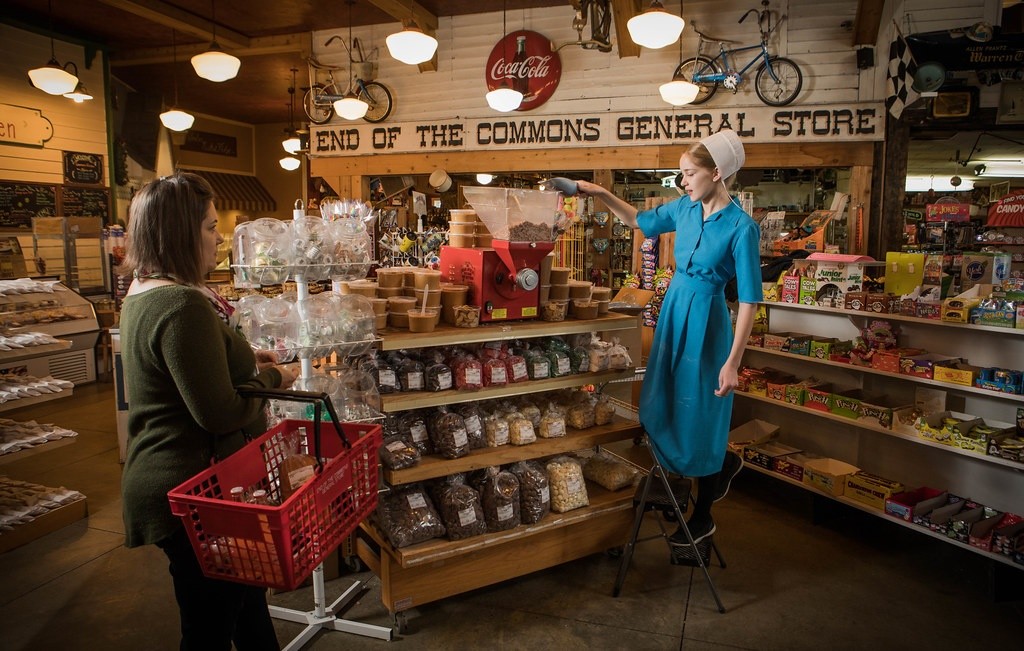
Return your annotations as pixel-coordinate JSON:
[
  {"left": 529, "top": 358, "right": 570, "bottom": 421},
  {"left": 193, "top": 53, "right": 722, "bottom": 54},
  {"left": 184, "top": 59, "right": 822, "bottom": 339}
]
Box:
[{"left": 0, "top": 185, "right": 1024, "bottom": 651}]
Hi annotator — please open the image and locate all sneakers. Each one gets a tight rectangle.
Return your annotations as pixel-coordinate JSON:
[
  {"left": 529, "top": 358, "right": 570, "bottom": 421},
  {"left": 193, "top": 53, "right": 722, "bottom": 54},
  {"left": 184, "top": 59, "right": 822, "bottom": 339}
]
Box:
[
  {"left": 713, "top": 451, "right": 744, "bottom": 503},
  {"left": 669, "top": 516, "right": 716, "bottom": 546}
]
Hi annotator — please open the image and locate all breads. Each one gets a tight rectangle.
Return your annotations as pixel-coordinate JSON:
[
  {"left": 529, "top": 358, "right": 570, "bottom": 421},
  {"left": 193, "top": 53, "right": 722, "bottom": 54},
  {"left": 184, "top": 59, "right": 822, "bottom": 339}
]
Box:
[{"left": 281, "top": 454, "right": 330, "bottom": 530}]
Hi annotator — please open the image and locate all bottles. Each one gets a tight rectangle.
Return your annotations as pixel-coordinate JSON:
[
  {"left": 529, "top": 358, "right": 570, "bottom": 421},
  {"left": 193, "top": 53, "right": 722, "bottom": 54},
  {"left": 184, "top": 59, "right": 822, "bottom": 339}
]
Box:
[
  {"left": 252, "top": 490, "right": 284, "bottom": 585},
  {"left": 110, "top": 225, "right": 124, "bottom": 253},
  {"left": 234, "top": 218, "right": 383, "bottom": 422},
  {"left": 230, "top": 486, "right": 261, "bottom": 578}
]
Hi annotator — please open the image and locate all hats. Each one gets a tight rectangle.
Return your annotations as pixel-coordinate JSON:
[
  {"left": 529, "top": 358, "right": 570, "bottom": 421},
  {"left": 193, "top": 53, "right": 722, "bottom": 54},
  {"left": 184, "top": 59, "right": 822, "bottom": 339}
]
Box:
[{"left": 699, "top": 130, "right": 745, "bottom": 182}]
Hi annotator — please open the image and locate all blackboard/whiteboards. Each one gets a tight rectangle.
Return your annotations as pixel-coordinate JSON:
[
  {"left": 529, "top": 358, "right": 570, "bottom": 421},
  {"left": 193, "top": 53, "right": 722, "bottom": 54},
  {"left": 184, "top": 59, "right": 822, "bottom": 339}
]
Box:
[
  {"left": 61, "top": 150, "right": 105, "bottom": 186},
  {"left": 0, "top": 179, "right": 113, "bottom": 233}
]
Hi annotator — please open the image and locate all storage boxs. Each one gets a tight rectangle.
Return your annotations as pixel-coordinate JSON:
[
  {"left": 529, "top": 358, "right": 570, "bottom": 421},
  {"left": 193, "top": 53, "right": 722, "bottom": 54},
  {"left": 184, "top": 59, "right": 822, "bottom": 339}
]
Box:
[
  {"left": 789, "top": 335, "right": 830, "bottom": 356},
  {"left": 726, "top": 419, "right": 780, "bottom": 457},
  {"left": 930, "top": 500, "right": 985, "bottom": 536},
  {"left": 743, "top": 440, "right": 803, "bottom": 471},
  {"left": 810, "top": 338, "right": 852, "bottom": 360},
  {"left": 784, "top": 376, "right": 831, "bottom": 406},
  {"left": 899, "top": 352, "right": 963, "bottom": 380},
  {"left": 933, "top": 363, "right": 984, "bottom": 387},
  {"left": 772, "top": 451, "right": 829, "bottom": 482},
  {"left": 843, "top": 470, "right": 905, "bottom": 510},
  {"left": 767, "top": 374, "right": 815, "bottom": 401},
  {"left": 912, "top": 492, "right": 965, "bottom": 528},
  {"left": 802, "top": 458, "right": 861, "bottom": 496},
  {"left": 763, "top": 332, "right": 807, "bottom": 352},
  {"left": 947, "top": 506, "right": 1005, "bottom": 544},
  {"left": 884, "top": 486, "right": 947, "bottom": 522},
  {"left": 872, "top": 346, "right": 928, "bottom": 374},
  {"left": 831, "top": 388, "right": 881, "bottom": 420},
  {"left": 803, "top": 382, "right": 857, "bottom": 413},
  {"left": 968, "top": 511, "right": 1024, "bottom": 552},
  {"left": 856, "top": 395, "right": 915, "bottom": 431}
]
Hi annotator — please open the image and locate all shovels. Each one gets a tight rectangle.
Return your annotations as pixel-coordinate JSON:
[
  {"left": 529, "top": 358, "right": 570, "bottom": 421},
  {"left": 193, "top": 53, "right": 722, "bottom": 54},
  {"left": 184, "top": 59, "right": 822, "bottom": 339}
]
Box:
[{"left": 538, "top": 177, "right": 579, "bottom": 198}]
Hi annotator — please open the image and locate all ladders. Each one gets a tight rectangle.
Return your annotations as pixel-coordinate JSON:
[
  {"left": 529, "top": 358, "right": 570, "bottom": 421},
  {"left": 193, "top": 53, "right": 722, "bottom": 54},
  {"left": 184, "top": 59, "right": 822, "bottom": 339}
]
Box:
[{"left": 613, "top": 436, "right": 726, "bottom": 614}]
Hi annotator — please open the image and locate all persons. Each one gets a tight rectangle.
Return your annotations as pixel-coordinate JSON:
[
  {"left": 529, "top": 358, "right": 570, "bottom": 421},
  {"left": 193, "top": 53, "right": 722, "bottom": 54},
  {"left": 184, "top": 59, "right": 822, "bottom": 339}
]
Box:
[
  {"left": 574, "top": 128, "right": 763, "bottom": 546},
  {"left": 119, "top": 173, "right": 302, "bottom": 651}
]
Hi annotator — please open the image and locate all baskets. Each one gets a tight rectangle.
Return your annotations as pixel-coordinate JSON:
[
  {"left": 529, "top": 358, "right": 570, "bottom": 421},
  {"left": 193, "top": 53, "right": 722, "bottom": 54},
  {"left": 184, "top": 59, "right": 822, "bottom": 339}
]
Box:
[{"left": 168, "top": 388, "right": 383, "bottom": 589}]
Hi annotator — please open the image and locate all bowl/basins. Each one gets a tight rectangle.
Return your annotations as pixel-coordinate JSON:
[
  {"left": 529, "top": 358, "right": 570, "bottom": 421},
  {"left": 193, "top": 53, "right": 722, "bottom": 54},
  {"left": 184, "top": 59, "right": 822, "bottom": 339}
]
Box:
[
  {"left": 448, "top": 209, "right": 492, "bottom": 248},
  {"left": 350, "top": 266, "right": 481, "bottom": 332},
  {"left": 542, "top": 266, "right": 611, "bottom": 323}
]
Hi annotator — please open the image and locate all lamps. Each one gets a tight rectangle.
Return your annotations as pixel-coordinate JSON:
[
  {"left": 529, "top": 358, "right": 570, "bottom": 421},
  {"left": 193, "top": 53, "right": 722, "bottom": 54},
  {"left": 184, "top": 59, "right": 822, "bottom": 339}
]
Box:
[
  {"left": 537, "top": 178, "right": 548, "bottom": 192},
  {"left": 332, "top": 0, "right": 368, "bottom": 121},
  {"left": 279, "top": 87, "right": 301, "bottom": 172},
  {"left": 190, "top": 0, "right": 241, "bottom": 83},
  {"left": 976, "top": 62, "right": 1006, "bottom": 87},
  {"left": 476, "top": 174, "right": 492, "bottom": 185},
  {"left": 385, "top": 0, "right": 439, "bottom": 66},
  {"left": 485, "top": 0, "right": 523, "bottom": 112},
  {"left": 26, "top": 0, "right": 79, "bottom": 96},
  {"left": 159, "top": 28, "right": 195, "bottom": 132},
  {"left": 282, "top": 68, "right": 309, "bottom": 156},
  {"left": 892, "top": 19, "right": 945, "bottom": 92},
  {"left": 626, "top": 0, "right": 685, "bottom": 49},
  {"left": 428, "top": 168, "right": 452, "bottom": 193},
  {"left": 63, "top": 80, "right": 94, "bottom": 104},
  {"left": 658, "top": 0, "right": 700, "bottom": 106}
]
